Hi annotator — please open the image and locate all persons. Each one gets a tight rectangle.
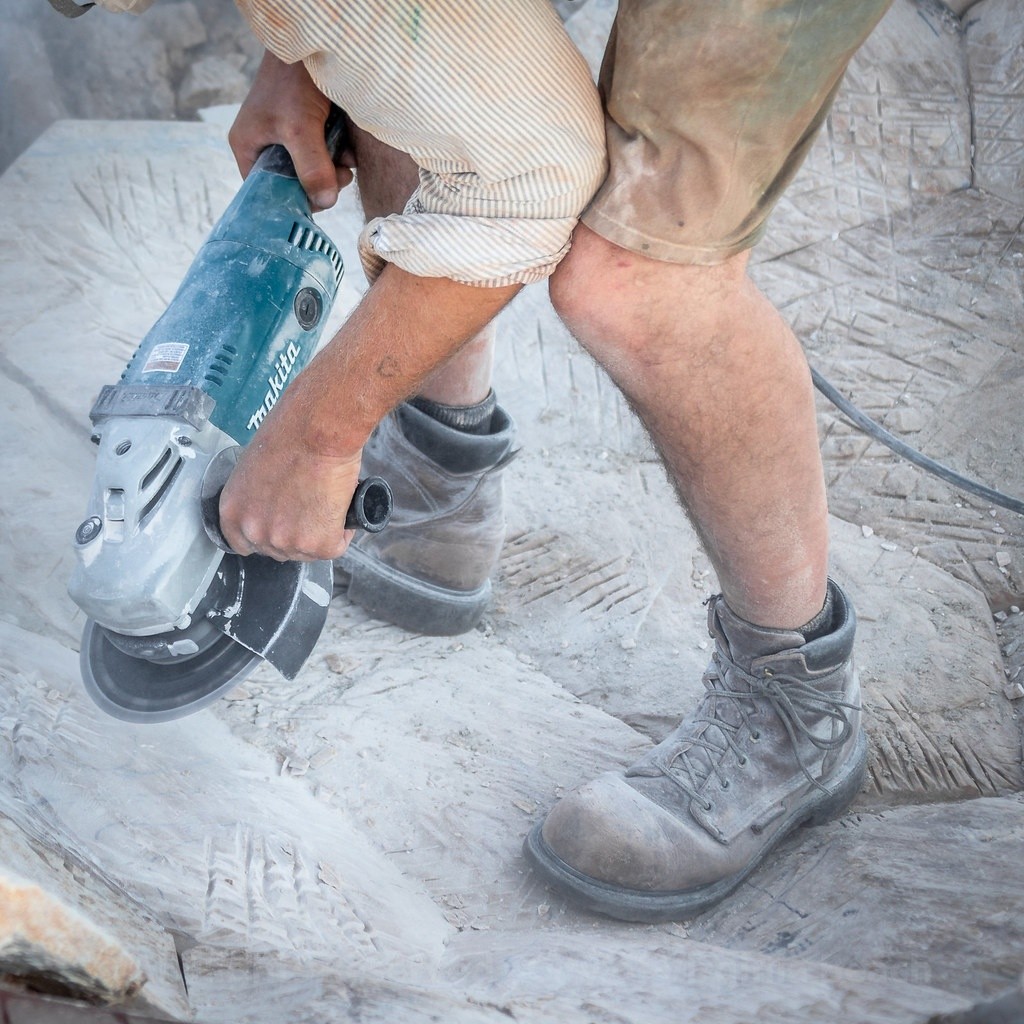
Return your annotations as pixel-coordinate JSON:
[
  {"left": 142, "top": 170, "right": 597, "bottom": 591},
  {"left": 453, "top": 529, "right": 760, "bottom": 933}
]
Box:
[{"left": 219, "top": 0, "right": 897, "bottom": 925}]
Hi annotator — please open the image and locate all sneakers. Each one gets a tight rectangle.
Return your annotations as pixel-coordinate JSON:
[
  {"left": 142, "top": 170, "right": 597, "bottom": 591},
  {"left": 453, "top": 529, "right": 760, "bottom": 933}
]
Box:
[
  {"left": 523, "top": 577, "right": 870, "bottom": 924},
  {"left": 333, "top": 399, "right": 523, "bottom": 636}
]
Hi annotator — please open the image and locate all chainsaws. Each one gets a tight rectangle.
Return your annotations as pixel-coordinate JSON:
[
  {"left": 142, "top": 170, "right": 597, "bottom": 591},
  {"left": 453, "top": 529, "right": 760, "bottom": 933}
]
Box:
[{"left": 60, "top": 101, "right": 336, "bottom": 727}]
